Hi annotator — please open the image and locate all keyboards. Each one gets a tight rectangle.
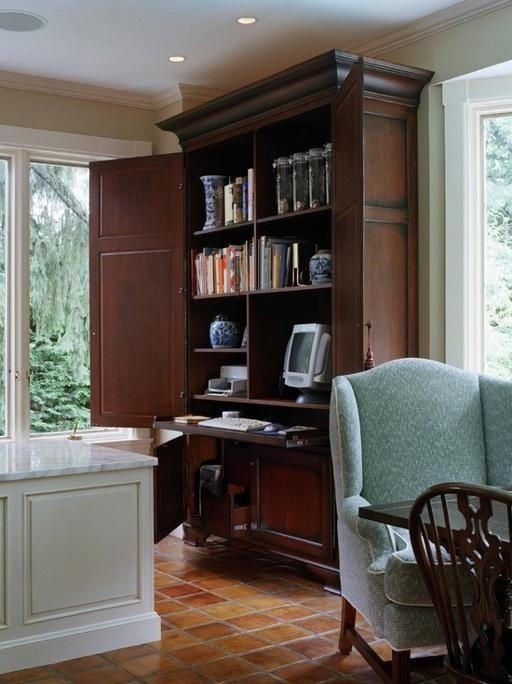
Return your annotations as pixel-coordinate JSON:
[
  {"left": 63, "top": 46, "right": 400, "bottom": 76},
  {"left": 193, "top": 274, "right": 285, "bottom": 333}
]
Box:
[{"left": 197, "top": 414, "right": 271, "bottom": 429}]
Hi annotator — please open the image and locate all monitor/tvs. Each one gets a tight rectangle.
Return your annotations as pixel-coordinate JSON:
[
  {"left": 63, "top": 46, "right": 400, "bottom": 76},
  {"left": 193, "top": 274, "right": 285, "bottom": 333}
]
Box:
[{"left": 283, "top": 320, "right": 332, "bottom": 403}]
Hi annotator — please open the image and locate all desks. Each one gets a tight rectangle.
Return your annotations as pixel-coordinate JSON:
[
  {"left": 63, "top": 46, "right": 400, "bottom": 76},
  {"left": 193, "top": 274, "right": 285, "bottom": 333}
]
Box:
[
  {"left": 1, "top": 440, "right": 164, "bottom": 674},
  {"left": 358, "top": 493, "right": 511, "bottom": 564}
]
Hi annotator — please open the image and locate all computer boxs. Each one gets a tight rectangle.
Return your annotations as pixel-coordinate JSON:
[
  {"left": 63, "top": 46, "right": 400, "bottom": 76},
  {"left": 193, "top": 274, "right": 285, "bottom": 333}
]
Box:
[{"left": 197, "top": 465, "right": 220, "bottom": 523}]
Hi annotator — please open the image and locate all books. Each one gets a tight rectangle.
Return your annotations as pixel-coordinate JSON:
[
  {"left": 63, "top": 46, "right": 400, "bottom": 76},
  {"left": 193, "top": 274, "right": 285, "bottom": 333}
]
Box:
[
  {"left": 174, "top": 415, "right": 210, "bottom": 424},
  {"left": 189, "top": 235, "right": 254, "bottom": 294},
  {"left": 257, "top": 235, "right": 306, "bottom": 290},
  {"left": 222, "top": 167, "right": 253, "bottom": 227}
]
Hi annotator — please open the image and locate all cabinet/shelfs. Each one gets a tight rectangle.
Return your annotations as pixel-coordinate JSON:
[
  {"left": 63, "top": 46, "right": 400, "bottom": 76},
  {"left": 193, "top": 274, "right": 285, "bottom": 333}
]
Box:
[
  {"left": 150, "top": 416, "right": 340, "bottom": 594},
  {"left": 89, "top": 49, "right": 435, "bottom": 428}
]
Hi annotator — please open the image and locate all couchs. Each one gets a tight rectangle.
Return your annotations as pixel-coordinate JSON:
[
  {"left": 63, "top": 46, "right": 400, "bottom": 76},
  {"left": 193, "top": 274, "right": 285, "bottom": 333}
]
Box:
[{"left": 330, "top": 356, "right": 511, "bottom": 684}]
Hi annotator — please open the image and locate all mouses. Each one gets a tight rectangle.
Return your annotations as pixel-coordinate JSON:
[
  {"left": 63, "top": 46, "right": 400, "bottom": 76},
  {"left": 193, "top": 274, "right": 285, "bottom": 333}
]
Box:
[{"left": 264, "top": 423, "right": 284, "bottom": 431}]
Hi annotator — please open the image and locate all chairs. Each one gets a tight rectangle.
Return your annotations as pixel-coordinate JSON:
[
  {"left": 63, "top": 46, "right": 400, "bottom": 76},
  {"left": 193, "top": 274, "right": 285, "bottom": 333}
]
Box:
[{"left": 409, "top": 483, "right": 512, "bottom": 684}]
